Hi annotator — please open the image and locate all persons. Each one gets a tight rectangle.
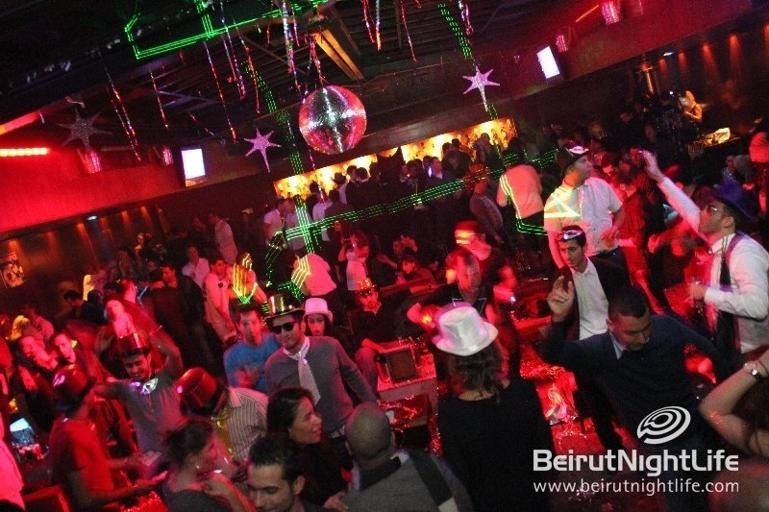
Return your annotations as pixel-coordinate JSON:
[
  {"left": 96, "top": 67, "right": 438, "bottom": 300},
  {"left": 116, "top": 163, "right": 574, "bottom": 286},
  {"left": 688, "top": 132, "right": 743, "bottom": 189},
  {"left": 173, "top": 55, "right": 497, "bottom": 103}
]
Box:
[
  {"left": 545, "top": 276, "right": 727, "bottom": 512},
  {"left": 431, "top": 95, "right": 769, "bottom": 307},
  {"left": 431, "top": 308, "right": 558, "bottom": 511},
  {"left": 336, "top": 400, "right": 474, "bottom": 512},
  {"left": 0, "top": 78, "right": 431, "bottom": 512},
  {"left": 699, "top": 345, "right": 769, "bottom": 463}
]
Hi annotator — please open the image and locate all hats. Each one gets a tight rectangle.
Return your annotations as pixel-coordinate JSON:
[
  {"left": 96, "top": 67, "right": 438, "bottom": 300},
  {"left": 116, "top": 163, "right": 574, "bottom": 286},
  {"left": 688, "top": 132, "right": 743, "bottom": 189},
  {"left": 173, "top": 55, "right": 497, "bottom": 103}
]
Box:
[
  {"left": 118, "top": 329, "right": 152, "bottom": 357},
  {"left": 264, "top": 292, "right": 304, "bottom": 322},
  {"left": 53, "top": 364, "right": 96, "bottom": 412},
  {"left": 332, "top": 172, "right": 346, "bottom": 185},
  {"left": 555, "top": 142, "right": 589, "bottom": 177},
  {"left": 356, "top": 278, "right": 376, "bottom": 290},
  {"left": 712, "top": 182, "right": 760, "bottom": 224},
  {"left": 435, "top": 307, "right": 498, "bottom": 355},
  {"left": 174, "top": 367, "right": 225, "bottom": 418},
  {"left": 302, "top": 296, "right": 333, "bottom": 323}
]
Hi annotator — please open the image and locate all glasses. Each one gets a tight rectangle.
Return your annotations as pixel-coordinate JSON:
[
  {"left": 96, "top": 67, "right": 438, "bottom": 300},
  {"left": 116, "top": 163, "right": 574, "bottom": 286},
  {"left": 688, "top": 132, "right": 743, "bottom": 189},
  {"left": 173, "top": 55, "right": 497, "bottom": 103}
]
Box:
[
  {"left": 271, "top": 321, "right": 295, "bottom": 334},
  {"left": 707, "top": 204, "right": 724, "bottom": 217}
]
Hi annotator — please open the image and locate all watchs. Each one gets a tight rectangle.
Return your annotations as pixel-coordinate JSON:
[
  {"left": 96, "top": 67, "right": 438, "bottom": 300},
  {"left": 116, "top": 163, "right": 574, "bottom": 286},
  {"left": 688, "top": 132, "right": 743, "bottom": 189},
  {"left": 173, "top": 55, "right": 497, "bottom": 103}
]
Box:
[{"left": 743, "top": 360, "right": 763, "bottom": 383}]
